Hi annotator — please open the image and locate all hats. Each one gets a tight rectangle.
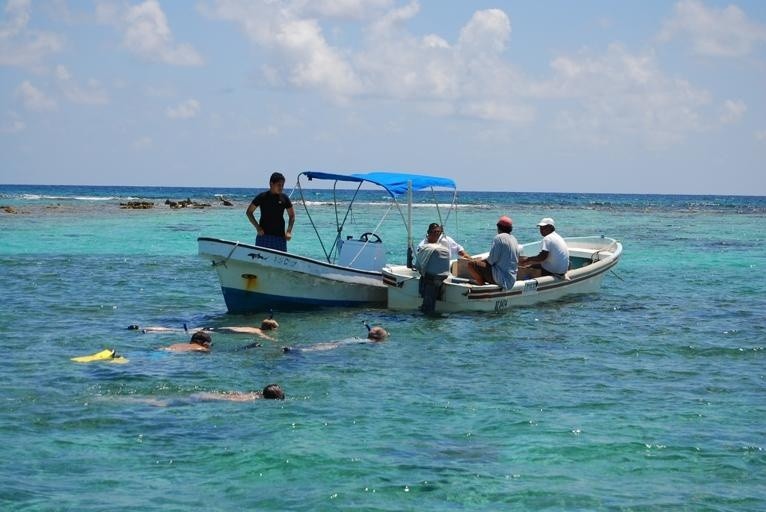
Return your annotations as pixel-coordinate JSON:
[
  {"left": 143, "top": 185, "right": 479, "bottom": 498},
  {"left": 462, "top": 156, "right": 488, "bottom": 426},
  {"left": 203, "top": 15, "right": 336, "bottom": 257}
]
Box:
[
  {"left": 499, "top": 216, "right": 512, "bottom": 227},
  {"left": 536, "top": 218, "right": 554, "bottom": 227}
]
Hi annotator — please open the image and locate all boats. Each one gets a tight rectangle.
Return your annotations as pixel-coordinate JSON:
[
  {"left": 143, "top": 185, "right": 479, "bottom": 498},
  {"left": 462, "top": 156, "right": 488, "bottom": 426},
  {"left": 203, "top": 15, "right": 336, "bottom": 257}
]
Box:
[
  {"left": 192, "top": 170, "right": 463, "bottom": 320},
  {"left": 378, "top": 228, "right": 623, "bottom": 317}
]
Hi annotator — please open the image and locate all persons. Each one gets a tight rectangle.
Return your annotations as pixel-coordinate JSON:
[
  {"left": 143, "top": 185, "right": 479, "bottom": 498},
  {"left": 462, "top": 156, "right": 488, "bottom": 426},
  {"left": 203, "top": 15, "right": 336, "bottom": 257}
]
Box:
[
  {"left": 465, "top": 216, "right": 520, "bottom": 290},
  {"left": 246, "top": 173, "right": 295, "bottom": 252},
  {"left": 126, "top": 318, "right": 389, "bottom": 407},
  {"left": 418, "top": 223, "right": 474, "bottom": 274},
  {"left": 519, "top": 216, "right": 570, "bottom": 276}
]
